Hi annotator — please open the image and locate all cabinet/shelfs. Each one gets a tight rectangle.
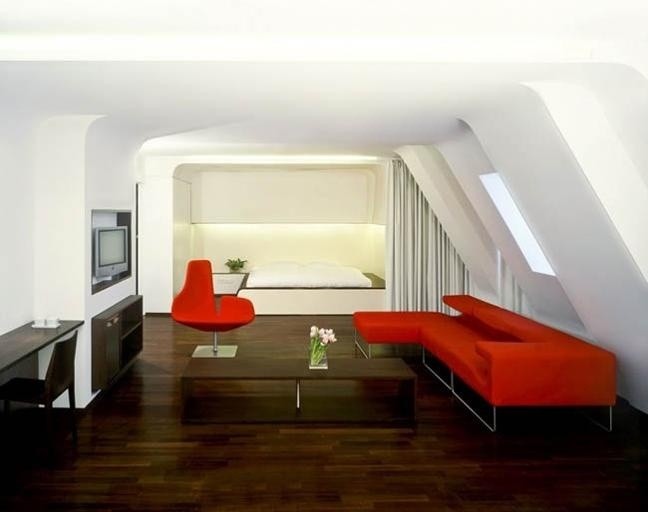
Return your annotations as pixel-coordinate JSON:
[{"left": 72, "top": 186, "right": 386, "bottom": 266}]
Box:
[{"left": 91, "top": 293, "right": 145, "bottom": 390}]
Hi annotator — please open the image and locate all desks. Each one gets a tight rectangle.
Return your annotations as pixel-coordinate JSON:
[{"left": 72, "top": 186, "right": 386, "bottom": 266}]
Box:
[{"left": 0, "top": 318, "right": 84, "bottom": 372}]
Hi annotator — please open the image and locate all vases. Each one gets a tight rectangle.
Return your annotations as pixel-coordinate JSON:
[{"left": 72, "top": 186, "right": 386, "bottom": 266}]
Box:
[{"left": 307, "top": 348, "right": 329, "bottom": 370}]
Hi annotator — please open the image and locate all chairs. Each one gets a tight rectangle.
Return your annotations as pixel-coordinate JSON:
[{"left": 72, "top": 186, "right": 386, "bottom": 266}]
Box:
[
  {"left": 171, "top": 259, "right": 257, "bottom": 357},
  {"left": 1, "top": 330, "right": 79, "bottom": 463}
]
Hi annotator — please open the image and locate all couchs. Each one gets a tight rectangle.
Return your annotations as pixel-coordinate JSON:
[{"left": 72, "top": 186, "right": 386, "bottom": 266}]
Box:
[{"left": 353, "top": 290, "right": 619, "bottom": 442}]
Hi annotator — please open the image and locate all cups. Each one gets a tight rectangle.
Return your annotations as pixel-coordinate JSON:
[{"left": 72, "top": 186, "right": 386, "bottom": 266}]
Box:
[{"left": 34, "top": 315, "right": 58, "bottom": 326}]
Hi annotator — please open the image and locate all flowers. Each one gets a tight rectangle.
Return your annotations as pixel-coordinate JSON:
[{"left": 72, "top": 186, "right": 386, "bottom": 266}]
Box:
[{"left": 308, "top": 322, "right": 337, "bottom": 362}]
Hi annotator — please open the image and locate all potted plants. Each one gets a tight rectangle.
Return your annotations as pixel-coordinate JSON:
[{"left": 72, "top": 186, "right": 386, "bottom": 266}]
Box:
[{"left": 224, "top": 258, "right": 248, "bottom": 273}]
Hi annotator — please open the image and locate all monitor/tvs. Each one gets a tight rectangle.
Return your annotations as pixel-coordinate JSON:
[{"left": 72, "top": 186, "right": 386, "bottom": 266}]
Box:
[{"left": 96, "top": 226, "right": 128, "bottom": 277}]
